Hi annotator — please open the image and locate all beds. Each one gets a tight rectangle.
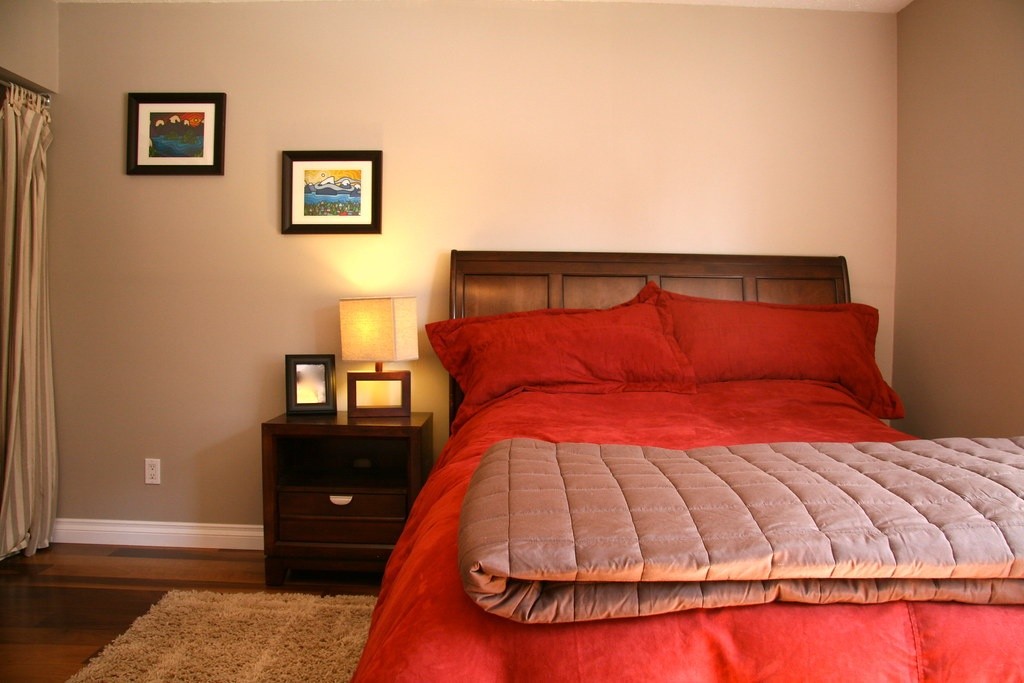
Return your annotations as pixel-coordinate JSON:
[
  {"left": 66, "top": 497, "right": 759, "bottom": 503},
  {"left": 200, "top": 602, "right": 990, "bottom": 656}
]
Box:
[{"left": 349, "top": 250, "right": 1024, "bottom": 682}]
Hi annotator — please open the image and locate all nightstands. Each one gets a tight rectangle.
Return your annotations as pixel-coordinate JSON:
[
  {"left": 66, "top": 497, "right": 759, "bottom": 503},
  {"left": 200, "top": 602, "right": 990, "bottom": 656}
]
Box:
[{"left": 261, "top": 413, "right": 432, "bottom": 587}]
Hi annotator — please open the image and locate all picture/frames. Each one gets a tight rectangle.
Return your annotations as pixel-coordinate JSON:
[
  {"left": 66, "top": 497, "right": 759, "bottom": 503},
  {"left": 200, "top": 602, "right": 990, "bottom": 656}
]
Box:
[
  {"left": 285, "top": 355, "right": 338, "bottom": 416},
  {"left": 281, "top": 150, "right": 382, "bottom": 234},
  {"left": 346, "top": 370, "right": 411, "bottom": 419},
  {"left": 127, "top": 92, "right": 226, "bottom": 176}
]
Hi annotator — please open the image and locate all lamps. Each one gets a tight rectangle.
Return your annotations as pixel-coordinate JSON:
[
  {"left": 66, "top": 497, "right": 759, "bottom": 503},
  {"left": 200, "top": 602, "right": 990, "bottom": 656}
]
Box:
[{"left": 339, "top": 297, "right": 419, "bottom": 372}]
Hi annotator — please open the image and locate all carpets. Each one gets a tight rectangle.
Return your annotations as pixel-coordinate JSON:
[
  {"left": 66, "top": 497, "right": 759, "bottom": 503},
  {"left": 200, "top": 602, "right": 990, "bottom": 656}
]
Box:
[{"left": 66, "top": 589, "right": 378, "bottom": 683}]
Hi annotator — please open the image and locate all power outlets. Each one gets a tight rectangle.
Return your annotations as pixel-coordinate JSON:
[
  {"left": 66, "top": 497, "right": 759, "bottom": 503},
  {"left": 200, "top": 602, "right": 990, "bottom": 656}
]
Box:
[{"left": 144, "top": 458, "right": 161, "bottom": 485}]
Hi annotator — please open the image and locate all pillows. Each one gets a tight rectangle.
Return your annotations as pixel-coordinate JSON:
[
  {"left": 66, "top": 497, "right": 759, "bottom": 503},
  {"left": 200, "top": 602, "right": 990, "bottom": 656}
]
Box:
[
  {"left": 664, "top": 290, "right": 905, "bottom": 421},
  {"left": 426, "top": 281, "right": 700, "bottom": 436}
]
